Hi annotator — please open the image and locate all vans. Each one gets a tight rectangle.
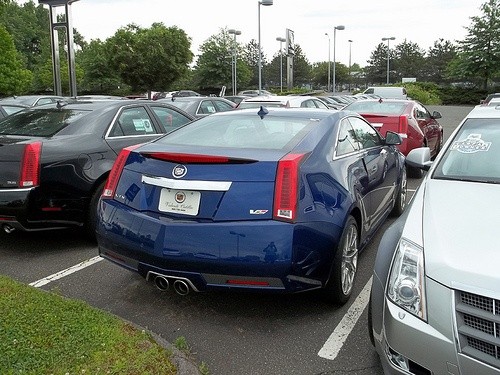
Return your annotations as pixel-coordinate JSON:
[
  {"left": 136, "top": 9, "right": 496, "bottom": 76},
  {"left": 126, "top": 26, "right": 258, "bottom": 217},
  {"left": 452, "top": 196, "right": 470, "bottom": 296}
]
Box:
[{"left": 361, "top": 86, "right": 408, "bottom": 100}]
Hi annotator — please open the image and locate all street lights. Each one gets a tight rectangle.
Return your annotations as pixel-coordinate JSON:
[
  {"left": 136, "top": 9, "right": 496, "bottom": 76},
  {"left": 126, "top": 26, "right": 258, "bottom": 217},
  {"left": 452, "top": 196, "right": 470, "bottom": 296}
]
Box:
[
  {"left": 225, "top": 55, "right": 234, "bottom": 97},
  {"left": 258, "top": 0, "right": 274, "bottom": 95},
  {"left": 325, "top": 33, "right": 330, "bottom": 93},
  {"left": 275, "top": 37, "right": 286, "bottom": 92},
  {"left": 348, "top": 39, "right": 353, "bottom": 92},
  {"left": 381, "top": 37, "right": 396, "bottom": 85},
  {"left": 333, "top": 25, "right": 345, "bottom": 96},
  {"left": 228, "top": 29, "right": 242, "bottom": 96},
  {"left": 37, "top": 0, "right": 79, "bottom": 97}
]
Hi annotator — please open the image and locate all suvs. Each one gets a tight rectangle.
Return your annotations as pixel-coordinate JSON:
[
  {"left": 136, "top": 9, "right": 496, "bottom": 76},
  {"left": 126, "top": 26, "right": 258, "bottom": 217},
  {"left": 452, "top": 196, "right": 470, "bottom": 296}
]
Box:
[{"left": 368, "top": 94, "right": 500, "bottom": 375}]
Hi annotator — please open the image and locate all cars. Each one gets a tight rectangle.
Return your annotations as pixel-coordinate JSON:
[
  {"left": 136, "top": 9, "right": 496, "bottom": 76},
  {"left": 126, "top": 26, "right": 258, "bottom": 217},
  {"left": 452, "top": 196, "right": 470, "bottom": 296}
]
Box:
[
  {"left": 0, "top": 99, "right": 198, "bottom": 241},
  {"left": 0, "top": 88, "right": 382, "bottom": 135},
  {"left": 95, "top": 106, "right": 408, "bottom": 305},
  {"left": 339, "top": 97, "right": 444, "bottom": 179}
]
